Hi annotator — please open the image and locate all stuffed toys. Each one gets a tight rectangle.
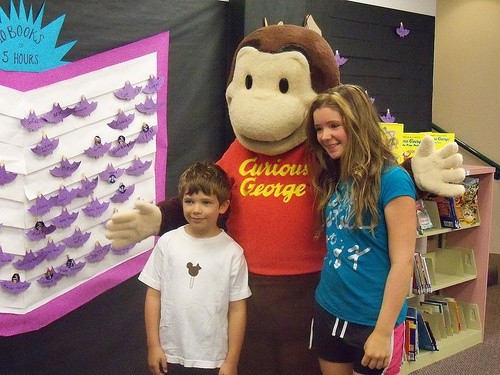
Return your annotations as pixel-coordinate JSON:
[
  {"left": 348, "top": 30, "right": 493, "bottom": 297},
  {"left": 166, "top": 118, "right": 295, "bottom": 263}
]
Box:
[{"left": 105, "top": 14, "right": 466, "bottom": 374}]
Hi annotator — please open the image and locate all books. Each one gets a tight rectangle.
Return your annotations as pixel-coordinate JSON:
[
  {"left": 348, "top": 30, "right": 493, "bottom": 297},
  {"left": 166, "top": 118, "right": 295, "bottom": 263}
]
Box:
[{"left": 403, "top": 177, "right": 479, "bottom": 364}]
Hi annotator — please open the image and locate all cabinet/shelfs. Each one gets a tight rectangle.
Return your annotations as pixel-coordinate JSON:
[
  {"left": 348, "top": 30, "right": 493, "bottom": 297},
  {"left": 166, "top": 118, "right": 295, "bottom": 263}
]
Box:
[{"left": 400, "top": 165, "right": 496, "bottom": 375}]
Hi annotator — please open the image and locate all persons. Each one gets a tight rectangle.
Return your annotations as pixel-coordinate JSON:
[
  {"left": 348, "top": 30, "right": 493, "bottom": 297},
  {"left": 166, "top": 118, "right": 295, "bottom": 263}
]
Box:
[
  {"left": 138, "top": 162, "right": 252, "bottom": 372},
  {"left": 308, "top": 84, "right": 416, "bottom": 372}
]
